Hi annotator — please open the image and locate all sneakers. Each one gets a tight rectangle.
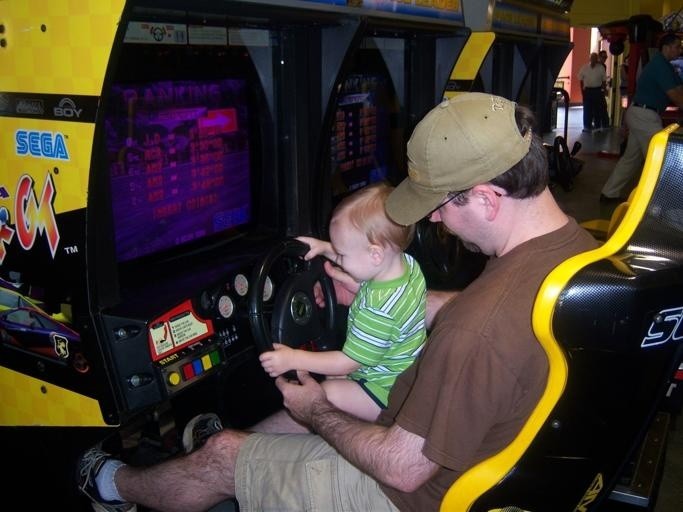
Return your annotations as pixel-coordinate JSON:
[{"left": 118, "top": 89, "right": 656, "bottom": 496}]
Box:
[
  {"left": 181, "top": 413, "right": 224, "bottom": 454},
  {"left": 75, "top": 447, "right": 137, "bottom": 512}
]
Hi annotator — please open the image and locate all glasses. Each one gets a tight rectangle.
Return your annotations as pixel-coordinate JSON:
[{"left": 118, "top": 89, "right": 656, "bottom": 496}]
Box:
[{"left": 414, "top": 187, "right": 502, "bottom": 225}]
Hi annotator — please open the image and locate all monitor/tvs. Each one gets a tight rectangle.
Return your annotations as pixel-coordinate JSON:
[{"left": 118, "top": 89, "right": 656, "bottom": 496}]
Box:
[
  {"left": 102, "top": 5, "right": 283, "bottom": 291},
  {"left": 327, "top": 26, "right": 415, "bottom": 208}
]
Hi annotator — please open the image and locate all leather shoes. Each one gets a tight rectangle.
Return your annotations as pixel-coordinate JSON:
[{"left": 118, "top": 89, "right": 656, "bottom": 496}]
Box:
[{"left": 599, "top": 193, "right": 623, "bottom": 204}]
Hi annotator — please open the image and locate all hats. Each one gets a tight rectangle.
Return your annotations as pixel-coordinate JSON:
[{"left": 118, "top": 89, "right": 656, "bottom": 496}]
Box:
[{"left": 383, "top": 91, "right": 533, "bottom": 227}]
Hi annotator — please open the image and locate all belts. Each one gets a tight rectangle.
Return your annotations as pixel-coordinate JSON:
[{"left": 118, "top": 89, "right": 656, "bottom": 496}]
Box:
[{"left": 632, "top": 102, "right": 657, "bottom": 114}]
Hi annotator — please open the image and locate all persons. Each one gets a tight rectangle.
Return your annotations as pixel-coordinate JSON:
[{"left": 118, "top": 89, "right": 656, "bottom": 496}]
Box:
[
  {"left": 600, "top": 34, "right": 683, "bottom": 202},
  {"left": 578, "top": 51, "right": 609, "bottom": 132}
]
